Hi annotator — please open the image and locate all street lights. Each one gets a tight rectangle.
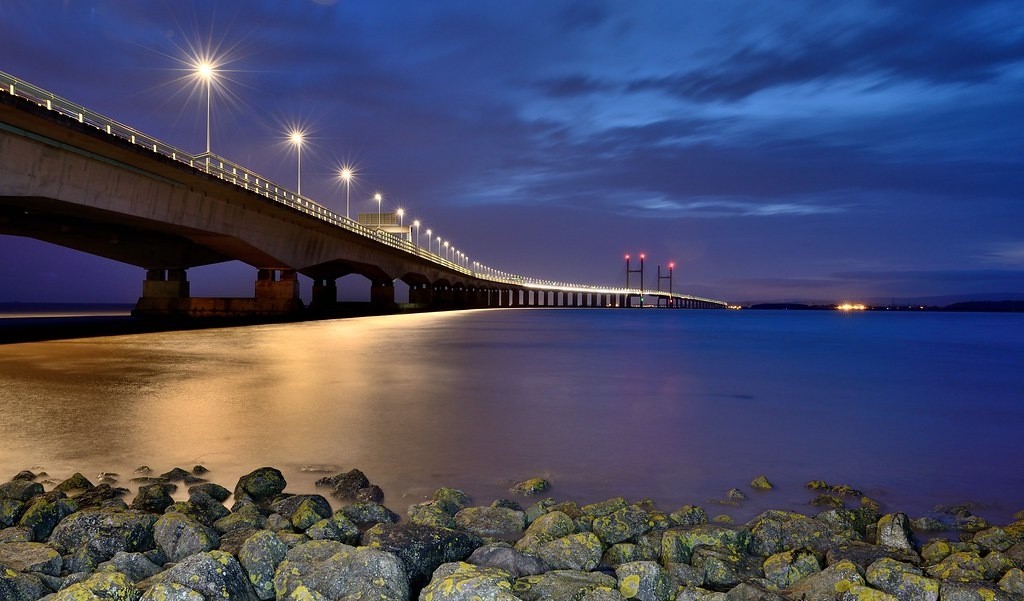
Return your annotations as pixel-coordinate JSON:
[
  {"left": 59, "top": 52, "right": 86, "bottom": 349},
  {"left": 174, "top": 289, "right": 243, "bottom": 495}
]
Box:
[
  {"left": 457, "top": 250, "right": 460, "bottom": 266},
  {"left": 414, "top": 220, "right": 419, "bottom": 247},
  {"left": 375, "top": 194, "right": 381, "bottom": 229},
  {"left": 451, "top": 247, "right": 454, "bottom": 264},
  {"left": 445, "top": 241, "right": 449, "bottom": 260},
  {"left": 398, "top": 209, "right": 404, "bottom": 239},
  {"left": 437, "top": 237, "right": 441, "bottom": 256},
  {"left": 461, "top": 253, "right": 464, "bottom": 268},
  {"left": 341, "top": 169, "right": 351, "bottom": 223},
  {"left": 202, "top": 61, "right": 214, "bottom": 162},
  {"left": 427, "top": 229, "right": 432, "bottom": 252},
  {"left": 465, "top": 257, "right": 468, "bottom": 271},
  {"left": 292, "top": 133, "right": 302, "bottom": 210},
  {"left": 477, "top": 261, "right": 560, "bottom": 287},
  {"left": 473, "top": 261, "right": 476, "bottom": 274}
]
